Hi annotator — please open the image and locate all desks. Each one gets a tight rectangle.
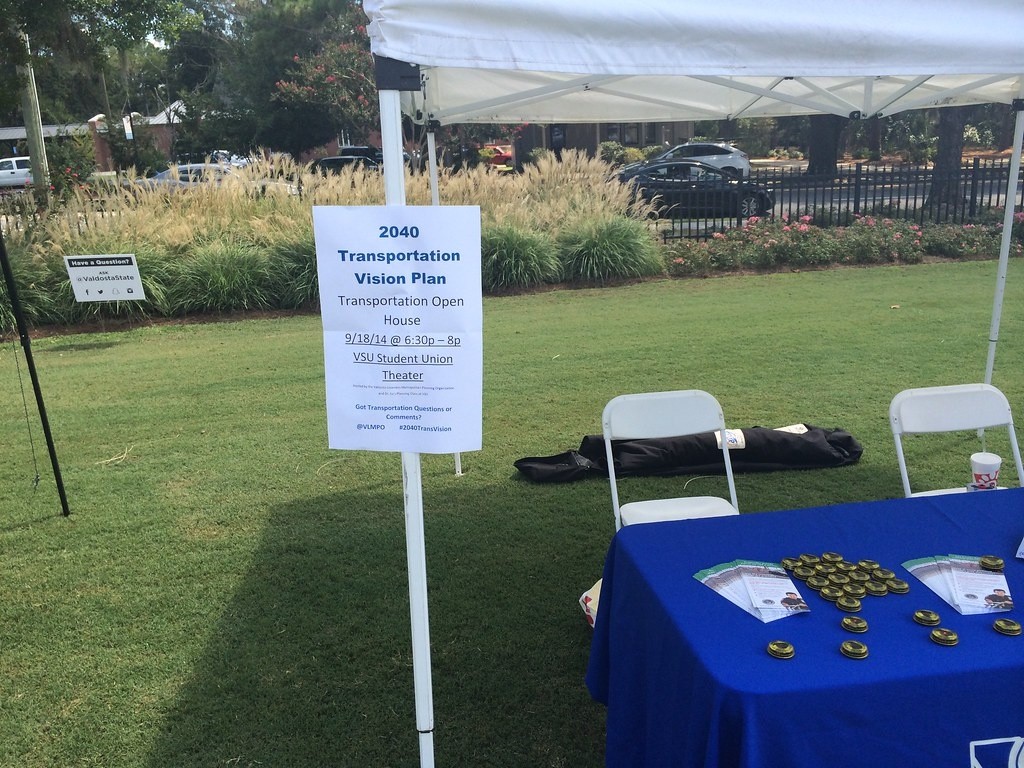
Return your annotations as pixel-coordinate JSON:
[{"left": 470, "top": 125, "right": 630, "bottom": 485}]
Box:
[{"left": 584, "top": 490, "right": 1024, "bottom": 768}]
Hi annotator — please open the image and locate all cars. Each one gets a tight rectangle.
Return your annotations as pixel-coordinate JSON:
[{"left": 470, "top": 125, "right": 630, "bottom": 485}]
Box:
[
  {"left": 657, "top": 141, "right": 750, "bottom": 178},
  {"left": 169, "top": 149, "right": 264, "bottom": 168},
  {"left": 134, "top": 164, "right": 301, "bottom": 197},
  {"left": 308, "top": 157, "right": 383, "bottom": 177},
  {"left": 477, "top": 144, "right": 512, "bottom": 165},
  {"left": 340, "top": 145, "right": 412, "bottom": 161},
  {"left": 604, "top": 159, "right": 776, "bottom": 219},
  {"left": 0, "top": 155, "right": 35, "bottom": 188}
]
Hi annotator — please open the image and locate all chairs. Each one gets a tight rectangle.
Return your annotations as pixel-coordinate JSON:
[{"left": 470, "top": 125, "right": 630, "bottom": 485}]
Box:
[
  {"left": 601, "top": 389, "right": 739, "bottom": 532},
  {"left": 889, "top": 384, "right": 1024, "bottom": 499}
]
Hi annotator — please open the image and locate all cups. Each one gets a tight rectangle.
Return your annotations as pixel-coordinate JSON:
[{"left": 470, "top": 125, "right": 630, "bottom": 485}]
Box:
[{"left": 971, "top": 453, "right": 1001, "bottom": 488}]
